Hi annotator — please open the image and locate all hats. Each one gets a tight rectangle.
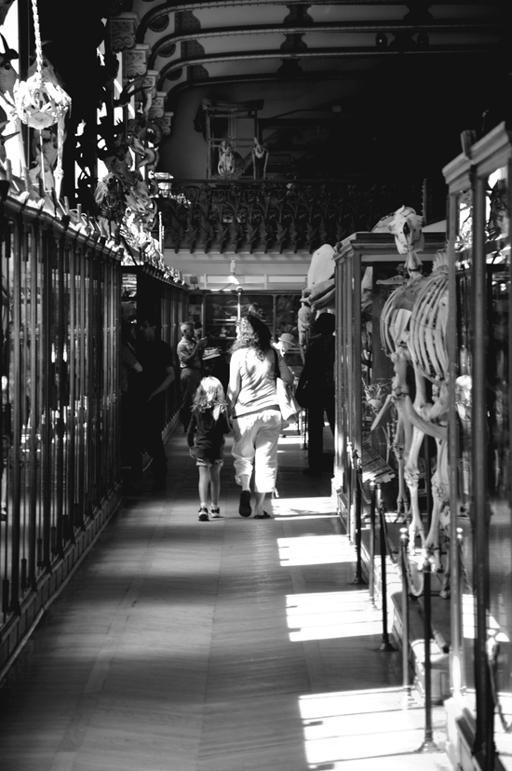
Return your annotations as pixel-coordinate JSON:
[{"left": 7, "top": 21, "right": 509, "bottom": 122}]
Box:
[{"left": 278, "top": 333, "right": 297, "bottom": 346}]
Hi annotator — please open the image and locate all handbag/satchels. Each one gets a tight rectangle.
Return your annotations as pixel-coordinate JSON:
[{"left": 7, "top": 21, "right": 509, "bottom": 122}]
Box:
[
  {"left": 276, "top": 377, "right": 302, "bottom": 421},
  {"left": 296, "top": 367, "right": 334, "bottom": 410}
]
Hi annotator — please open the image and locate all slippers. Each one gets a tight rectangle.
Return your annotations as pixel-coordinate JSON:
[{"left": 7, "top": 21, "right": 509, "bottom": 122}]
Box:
[
  {"left": 238, "top": 490, "right": 251, "bottom": 517},
  {"left": 252, "top": 510, "right": 270, "bottom": 518}
]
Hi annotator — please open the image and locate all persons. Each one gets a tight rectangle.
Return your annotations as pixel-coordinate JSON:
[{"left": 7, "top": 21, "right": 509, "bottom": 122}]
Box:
[
  {"left": 123, "top": 312, "right": 336, "bottom": 476},
  {"left": 186, "top": 313, "right": 294, "bottom": 521}
]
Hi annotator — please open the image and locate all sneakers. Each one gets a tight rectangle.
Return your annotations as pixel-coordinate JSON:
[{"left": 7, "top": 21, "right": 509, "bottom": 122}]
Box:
[
  {"left": 210, "top": 507, "right": 220, "bottom": 518},
  {"left": 198, "top": 508, "right": 209, "bottom": 521}
]
